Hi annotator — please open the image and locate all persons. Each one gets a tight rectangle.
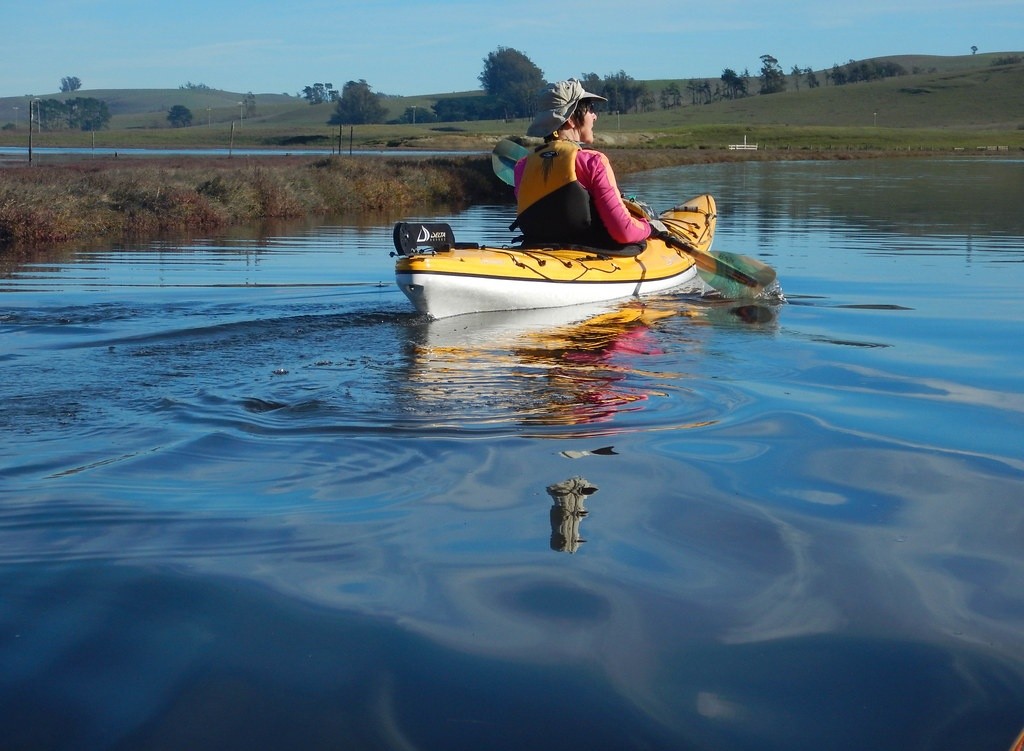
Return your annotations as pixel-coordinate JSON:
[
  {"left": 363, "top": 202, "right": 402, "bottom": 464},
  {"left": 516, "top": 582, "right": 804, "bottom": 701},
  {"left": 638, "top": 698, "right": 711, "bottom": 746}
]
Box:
[{"left": 515, "top": 78, "right": 672, "bottom": 246}]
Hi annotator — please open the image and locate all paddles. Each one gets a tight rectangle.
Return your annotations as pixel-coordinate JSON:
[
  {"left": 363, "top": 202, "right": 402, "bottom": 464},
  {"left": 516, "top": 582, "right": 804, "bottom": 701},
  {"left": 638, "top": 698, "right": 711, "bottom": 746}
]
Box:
[{"left": 492, "top": 134, "right": 779, "bottom": 301}]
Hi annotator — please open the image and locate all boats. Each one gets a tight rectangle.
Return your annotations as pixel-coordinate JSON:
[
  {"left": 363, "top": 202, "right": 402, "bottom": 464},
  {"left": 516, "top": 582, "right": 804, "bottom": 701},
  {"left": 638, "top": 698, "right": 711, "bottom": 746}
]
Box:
[{"left": 395, "top": 195, "right": 716, "bottom": 320}]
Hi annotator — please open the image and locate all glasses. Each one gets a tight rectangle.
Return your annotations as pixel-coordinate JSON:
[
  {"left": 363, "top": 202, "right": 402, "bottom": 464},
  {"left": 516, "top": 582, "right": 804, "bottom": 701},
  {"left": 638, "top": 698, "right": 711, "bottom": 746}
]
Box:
[{"left": 586, "top": 103, "right": 595, "bottom": 114}]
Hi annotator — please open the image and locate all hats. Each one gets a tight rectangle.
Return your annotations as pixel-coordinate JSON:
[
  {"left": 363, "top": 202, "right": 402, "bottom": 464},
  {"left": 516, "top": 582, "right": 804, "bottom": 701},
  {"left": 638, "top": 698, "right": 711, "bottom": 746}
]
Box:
[{"left": 526, "top": 77, "right": 608, "bottom": 138}]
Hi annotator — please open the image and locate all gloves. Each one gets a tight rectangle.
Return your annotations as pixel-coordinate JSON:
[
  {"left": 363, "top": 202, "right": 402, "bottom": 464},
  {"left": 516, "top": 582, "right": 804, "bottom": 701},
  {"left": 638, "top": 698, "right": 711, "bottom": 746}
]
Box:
[{"left": 648, "top": 220, "right": 669, "bottom": 237}]
